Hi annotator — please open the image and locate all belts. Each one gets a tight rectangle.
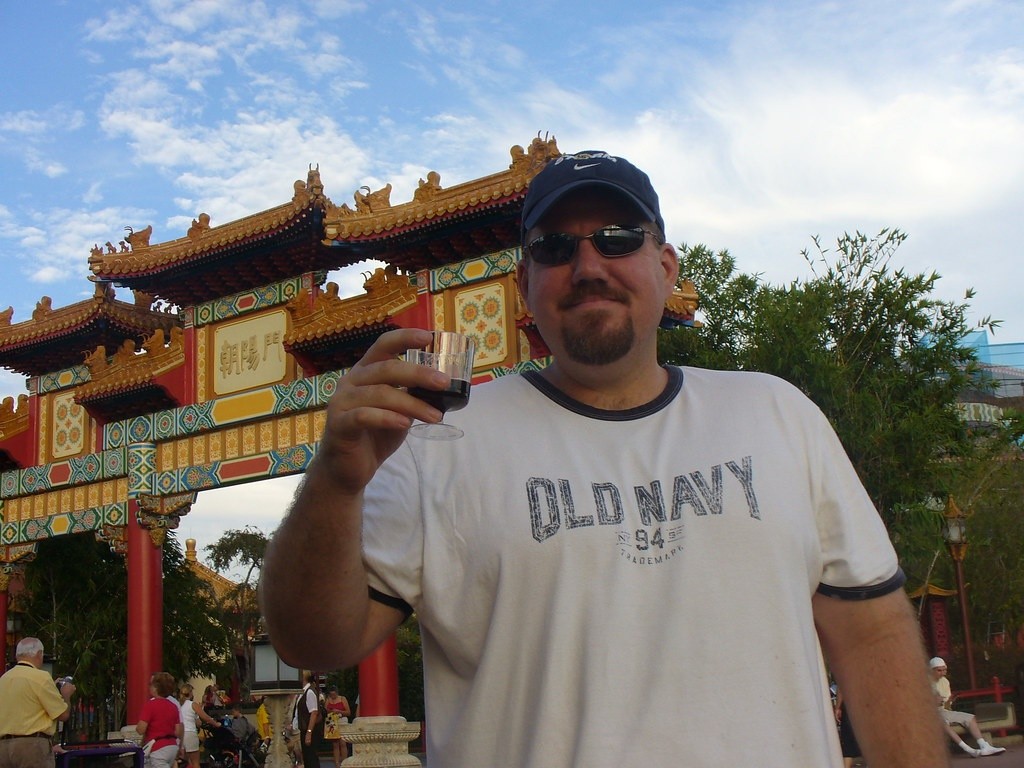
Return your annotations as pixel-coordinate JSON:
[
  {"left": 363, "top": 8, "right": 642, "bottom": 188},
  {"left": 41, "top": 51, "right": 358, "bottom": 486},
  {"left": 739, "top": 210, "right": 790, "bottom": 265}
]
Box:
[{"left": 0, "top": 732, "right": 53, "bottom": 740}]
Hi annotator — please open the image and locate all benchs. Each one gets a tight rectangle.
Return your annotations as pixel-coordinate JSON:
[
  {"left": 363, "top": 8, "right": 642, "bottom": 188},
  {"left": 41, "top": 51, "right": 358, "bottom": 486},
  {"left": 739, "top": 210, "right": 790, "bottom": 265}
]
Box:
[{"left": 951, "top": 703, "right": 1017, "bottom": 747}]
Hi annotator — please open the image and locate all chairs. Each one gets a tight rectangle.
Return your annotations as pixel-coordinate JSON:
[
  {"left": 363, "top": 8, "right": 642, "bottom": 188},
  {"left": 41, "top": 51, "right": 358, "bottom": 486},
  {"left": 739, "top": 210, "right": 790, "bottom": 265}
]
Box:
[{"left": 234, "top": 724, "right": 259, "bottom": 768}]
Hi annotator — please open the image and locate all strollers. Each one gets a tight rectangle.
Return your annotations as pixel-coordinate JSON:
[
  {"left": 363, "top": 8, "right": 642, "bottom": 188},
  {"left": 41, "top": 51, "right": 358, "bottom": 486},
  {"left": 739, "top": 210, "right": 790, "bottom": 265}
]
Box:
[
  {"left": 200, "top": 719, "right": 261, "bottom": 768},
  {"left": 53, "top": 739, "right": 145, "bottom": 768}
]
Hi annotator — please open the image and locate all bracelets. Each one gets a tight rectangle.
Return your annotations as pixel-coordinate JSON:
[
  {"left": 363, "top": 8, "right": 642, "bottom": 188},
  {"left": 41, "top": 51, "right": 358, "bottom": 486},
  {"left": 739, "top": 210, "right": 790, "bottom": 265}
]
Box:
[{"left": 265, "top": 736, "right": 270, "bottom": 739}]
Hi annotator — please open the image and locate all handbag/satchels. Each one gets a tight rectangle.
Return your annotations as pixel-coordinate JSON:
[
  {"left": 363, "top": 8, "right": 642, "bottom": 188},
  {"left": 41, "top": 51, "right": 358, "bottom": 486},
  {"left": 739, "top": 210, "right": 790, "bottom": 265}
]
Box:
[{"left": 323, "top": 712, "right": 341, "bottom": 739}]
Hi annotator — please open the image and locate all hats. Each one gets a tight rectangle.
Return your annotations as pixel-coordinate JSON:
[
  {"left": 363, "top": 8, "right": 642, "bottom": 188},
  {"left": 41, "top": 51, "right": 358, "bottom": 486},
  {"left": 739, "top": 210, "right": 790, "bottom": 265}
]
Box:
[
  {"left": 930, "top": 656, "right": 945, "bottom": 670},
  {"left": 524, "top": 150, "right": 665, "bottom": 242}
]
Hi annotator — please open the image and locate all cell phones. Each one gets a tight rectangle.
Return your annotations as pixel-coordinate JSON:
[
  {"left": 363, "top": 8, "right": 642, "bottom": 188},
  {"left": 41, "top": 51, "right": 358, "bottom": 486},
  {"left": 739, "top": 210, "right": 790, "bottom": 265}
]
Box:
[{"left": 952, "top": 691, "right": 960, "bottom": 702}]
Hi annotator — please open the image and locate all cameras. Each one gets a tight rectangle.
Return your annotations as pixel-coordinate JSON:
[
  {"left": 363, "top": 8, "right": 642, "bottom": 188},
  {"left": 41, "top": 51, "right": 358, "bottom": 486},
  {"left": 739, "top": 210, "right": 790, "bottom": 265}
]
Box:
[{"left": 58, "top": 676, "right": 73, "bottom": 688}]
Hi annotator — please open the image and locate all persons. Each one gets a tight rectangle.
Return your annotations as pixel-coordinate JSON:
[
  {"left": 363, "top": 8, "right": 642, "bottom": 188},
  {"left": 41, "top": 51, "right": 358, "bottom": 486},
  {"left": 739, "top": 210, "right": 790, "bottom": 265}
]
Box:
[
  {"left": 829, "top": 672, "right": 868, "bottom": 768},
  {"left": 177, "top": 684, "right": 222, "bottom": 768},
  {"left": 323, "top": 685, "right": 351, "bottom": 768},
  {"left": 286, "top": 671, "right": 321, "bottom": 768},
  {"left": 261, "top": 150, "right": 953, "bottom": 768},
  {"left": 0, "top": 637, "right": 76, "bottom": 768},
  {"left": 232, "top": 707, "right": 249, "bottom": 747},
  {"left": 928, "top": 656, "right": 1006, "bottom": 757},
  {"left": 202, "top": 684, "right": 228, "bottom": 710},
  {"left": 354, "top": 692, "right": 360, "bottom": 717},
  {"left": 136, "top": 670, "right": 184, "bottom": 768},
  {"left": 256, "top": 696, "right": 273, "bottom": 754}
]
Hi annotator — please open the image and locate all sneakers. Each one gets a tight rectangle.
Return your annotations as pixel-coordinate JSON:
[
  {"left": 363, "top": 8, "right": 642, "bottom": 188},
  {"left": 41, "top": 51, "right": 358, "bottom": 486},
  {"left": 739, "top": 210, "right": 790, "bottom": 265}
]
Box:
[
  {"left": 970, "top": 749, "right": 981, "bottom": 757},
  {"left": 980, "top": 743, "right": 1006, "bottom": 756}
]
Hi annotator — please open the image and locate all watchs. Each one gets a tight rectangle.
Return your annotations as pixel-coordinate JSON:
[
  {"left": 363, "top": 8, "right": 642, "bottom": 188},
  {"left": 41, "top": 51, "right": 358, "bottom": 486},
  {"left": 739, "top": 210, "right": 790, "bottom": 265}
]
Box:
[{"left": 306, "top": 729, "right": 312, "bottom": 733}]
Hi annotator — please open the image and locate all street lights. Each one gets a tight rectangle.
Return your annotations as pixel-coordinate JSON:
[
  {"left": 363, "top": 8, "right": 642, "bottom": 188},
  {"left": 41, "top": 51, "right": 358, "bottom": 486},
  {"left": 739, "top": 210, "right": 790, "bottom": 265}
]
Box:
[{"left": 938, "top": 493, "right": 982, "bottom": 708}]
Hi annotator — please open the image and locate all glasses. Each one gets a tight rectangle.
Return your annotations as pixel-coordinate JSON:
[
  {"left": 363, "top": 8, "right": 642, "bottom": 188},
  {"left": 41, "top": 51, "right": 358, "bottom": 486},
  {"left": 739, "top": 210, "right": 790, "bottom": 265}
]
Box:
[{"left": 520, "top": 223, "right": 665, "bottom": 265}]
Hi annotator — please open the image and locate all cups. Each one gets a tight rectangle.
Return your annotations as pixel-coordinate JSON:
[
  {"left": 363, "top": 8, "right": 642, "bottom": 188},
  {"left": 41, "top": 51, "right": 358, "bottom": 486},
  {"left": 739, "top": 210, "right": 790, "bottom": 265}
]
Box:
[{"left": 406, "top": 330, "right": 474, "bottom": 411}]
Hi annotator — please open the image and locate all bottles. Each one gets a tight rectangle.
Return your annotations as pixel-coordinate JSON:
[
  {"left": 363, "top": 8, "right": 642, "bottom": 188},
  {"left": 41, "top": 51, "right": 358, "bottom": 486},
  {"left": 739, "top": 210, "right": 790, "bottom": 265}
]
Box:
[{"left": 224, "top": 715, "right": 229, "bottom": 726}]
